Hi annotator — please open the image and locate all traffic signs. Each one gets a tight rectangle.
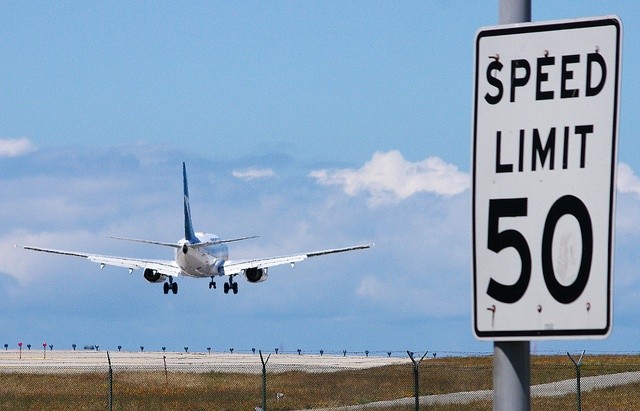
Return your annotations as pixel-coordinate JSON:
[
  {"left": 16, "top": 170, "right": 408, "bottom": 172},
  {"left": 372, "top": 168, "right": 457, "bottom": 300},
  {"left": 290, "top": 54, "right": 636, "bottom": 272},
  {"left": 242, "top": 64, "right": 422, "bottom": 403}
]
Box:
[{"left": 471, "top": 15, "right": 622, "bottom": 343}]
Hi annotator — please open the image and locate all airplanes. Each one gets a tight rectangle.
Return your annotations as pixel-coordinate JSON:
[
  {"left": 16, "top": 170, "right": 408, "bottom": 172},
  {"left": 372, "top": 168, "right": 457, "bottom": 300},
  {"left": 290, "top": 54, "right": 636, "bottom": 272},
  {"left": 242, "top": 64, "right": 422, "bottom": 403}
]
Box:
[{"left": 21, "top": 162, "right": 374, "bottom": 296}]
